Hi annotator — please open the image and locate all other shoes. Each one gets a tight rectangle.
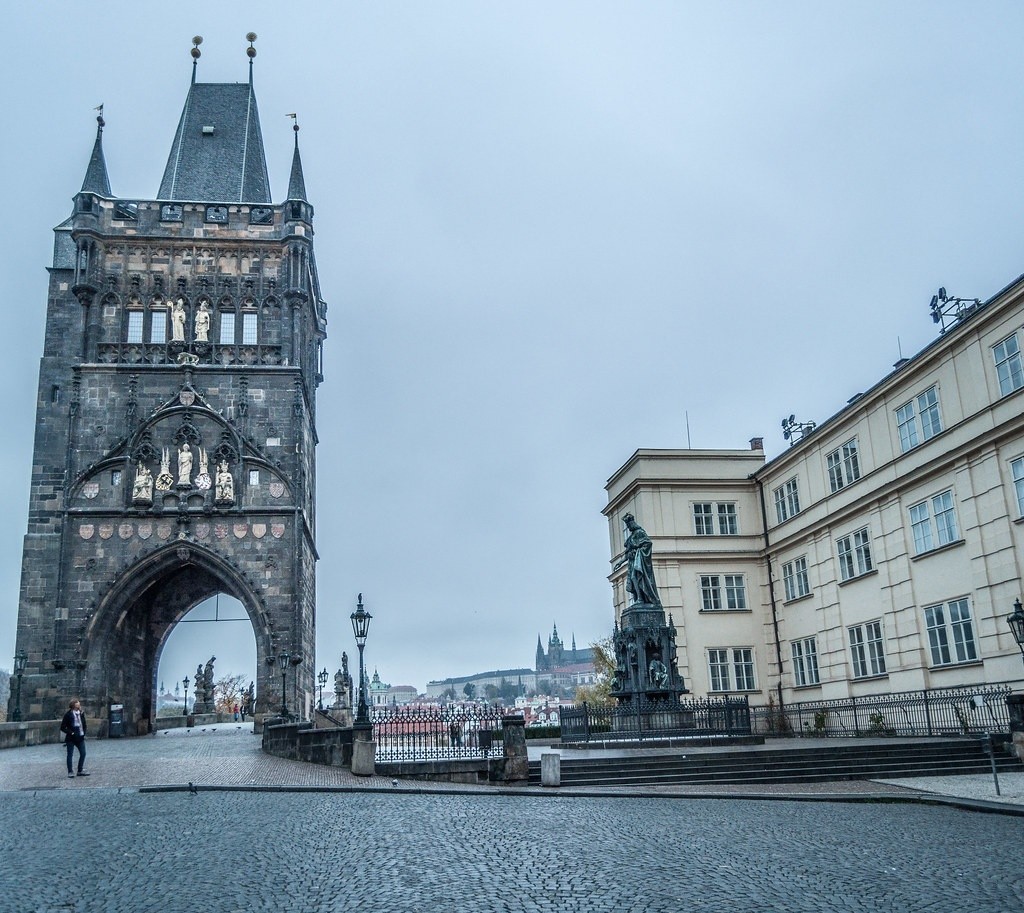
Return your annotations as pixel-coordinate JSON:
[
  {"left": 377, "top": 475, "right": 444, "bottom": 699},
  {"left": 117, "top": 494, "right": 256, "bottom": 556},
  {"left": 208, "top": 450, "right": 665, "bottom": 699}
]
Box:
[
  {"left": 77, "top": 771, "right": 90, "bottom": 776},
  {"left": 68, "top": 773, "right": 75, "bottom": 778}
]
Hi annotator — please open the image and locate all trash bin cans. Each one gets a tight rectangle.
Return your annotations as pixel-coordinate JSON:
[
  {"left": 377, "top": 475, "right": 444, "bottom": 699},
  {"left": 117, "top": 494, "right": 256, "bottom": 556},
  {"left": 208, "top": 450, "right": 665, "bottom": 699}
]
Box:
[{"left": 109, "top": 703, "right": 124, "bottom": 738}]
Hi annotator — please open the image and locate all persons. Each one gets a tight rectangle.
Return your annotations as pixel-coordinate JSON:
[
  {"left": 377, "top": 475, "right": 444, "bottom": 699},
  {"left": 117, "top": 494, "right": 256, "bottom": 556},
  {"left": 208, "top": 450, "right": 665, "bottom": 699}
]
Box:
[
  {"left": 194, "top": 301, "right": 210, "bottom": 340},
  {"left": 133, "top": 467, "right": 152, "bottom": 499},
  {"left": 215, "top": 461, "right": 232, "bottom": 498},
  {"left": 614, "top": 514, "right": 653, "bottom": 605},
  {"left": 249, "top": 681, "right": 255, "bottom": 693},
  {"left": 204, "top": 656, "right": 216, "bottom": 682},
  {"left": 234, "top": 705, "right": 239, "bottom": 721},
  {"left": 172, "top": 299, "right": 186, "bottom": 340},
  {"left": 196, "top": 664, "right": 203, "bottom": 680},
  {"left": 240, "top": 704, "right": 247, "bottom": 721},
  {"left": 649, "top": 653, "right": 669, "bottom": 689},
  {"left": 178, "top": 444, "right": 192, "bottom": 485},
  {"left": 60, "top": 698, "right": 90, "bottom": 779}
]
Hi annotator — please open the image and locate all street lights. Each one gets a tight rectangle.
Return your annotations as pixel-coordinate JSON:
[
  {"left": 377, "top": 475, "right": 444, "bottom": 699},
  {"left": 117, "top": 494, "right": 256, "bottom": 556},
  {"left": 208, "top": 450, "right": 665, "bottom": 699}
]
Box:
[
  {"left": 318, "top": 666, "right": 329, "bottom": 710},
  {"left": 182, "top": 676, "right": 190, "bottom": 716},
  {"left": 240, "top": 687, "right": 245, "bottom": 705},
  {"left": 1003, "top": 598, "right": 1024, "bottom": 663},
  {"left": 346, "top": 594, "right": 373, "bottom": 729},
  {"left": 278, "top": 648, "right": 290, "bottom": 717},
  {"left": 12, "top": 647, "right": 27, "bottom": 722}
]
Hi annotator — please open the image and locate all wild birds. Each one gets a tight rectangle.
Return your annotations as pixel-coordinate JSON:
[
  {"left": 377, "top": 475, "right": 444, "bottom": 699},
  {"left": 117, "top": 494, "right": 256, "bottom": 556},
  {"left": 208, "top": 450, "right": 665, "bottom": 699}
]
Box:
[
  {"left": 164, "top": 726, "right": 253, "bottom": 734},
  {"left": 188, "top": 781, "right": 198, "bottom": 795},
  {"left": 392, "top": 778, "right": 398, "bottom": 787}
]
